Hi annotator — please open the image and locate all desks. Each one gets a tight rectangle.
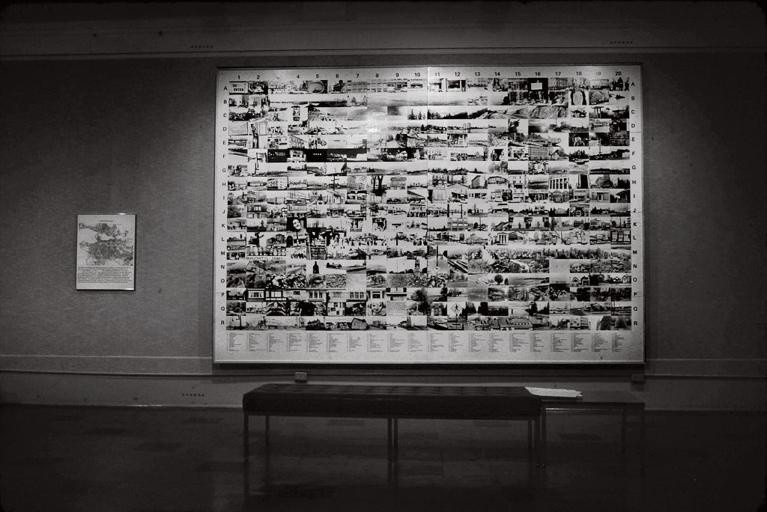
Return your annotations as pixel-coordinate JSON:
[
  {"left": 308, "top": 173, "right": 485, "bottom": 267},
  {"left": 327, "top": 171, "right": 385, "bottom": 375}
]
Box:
[{"left": 241, "top": 383, "right": 645, "bottom": 504}]
